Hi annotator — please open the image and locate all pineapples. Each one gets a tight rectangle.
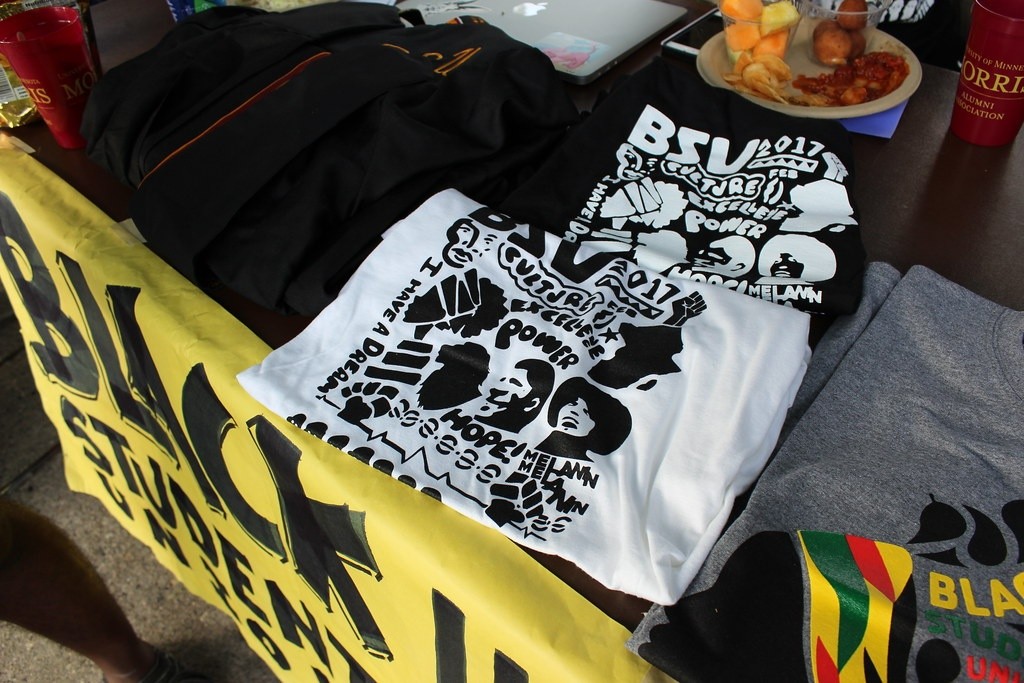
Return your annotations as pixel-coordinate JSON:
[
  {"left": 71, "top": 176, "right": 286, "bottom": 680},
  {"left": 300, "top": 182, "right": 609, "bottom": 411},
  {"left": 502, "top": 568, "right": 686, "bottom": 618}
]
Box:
[{"left": 761, "top": 0, "right": 801, "bottom": 38}]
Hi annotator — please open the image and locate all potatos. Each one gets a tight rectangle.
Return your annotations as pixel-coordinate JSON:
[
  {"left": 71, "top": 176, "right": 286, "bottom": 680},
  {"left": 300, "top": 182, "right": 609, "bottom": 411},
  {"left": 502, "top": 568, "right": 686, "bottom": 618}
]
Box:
[{"left": 812, "top": 0, "right": 869, "bottom": 65}]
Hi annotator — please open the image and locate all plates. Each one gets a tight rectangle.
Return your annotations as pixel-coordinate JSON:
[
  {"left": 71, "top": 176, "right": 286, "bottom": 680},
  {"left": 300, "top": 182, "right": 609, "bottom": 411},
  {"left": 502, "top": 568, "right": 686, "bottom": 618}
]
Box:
[{"left": 696, "top": 14, "right": 922, "bottom": 119}]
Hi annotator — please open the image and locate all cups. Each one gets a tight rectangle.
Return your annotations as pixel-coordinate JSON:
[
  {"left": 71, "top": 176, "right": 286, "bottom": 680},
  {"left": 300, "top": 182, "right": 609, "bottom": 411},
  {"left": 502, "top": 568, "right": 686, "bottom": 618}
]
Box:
[
  {"left": 0, "top": 6, "right": 99, "bottom": 151},
  {"left": 950, "top": 0, "right": 1024, "bottom": 146},
  {"left": 716, "top": 0, "right": 805, "bottom": 72},
  {"left": 805, "top": 0, "right": 894, "bottom": 67}
]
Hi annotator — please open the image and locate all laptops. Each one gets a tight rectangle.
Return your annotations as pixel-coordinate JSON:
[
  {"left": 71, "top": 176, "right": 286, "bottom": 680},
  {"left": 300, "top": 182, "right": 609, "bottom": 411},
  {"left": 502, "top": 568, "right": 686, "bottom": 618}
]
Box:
[{"left": 393, "top": 0, "right": 687, "bottom": 85}]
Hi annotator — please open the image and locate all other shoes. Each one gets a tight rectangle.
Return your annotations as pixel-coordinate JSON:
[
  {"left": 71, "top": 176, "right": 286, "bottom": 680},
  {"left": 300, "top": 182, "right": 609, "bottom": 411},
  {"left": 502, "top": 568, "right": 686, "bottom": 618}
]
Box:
[{"left": 104, "top": 640, "right": 186, "bottom": 683}]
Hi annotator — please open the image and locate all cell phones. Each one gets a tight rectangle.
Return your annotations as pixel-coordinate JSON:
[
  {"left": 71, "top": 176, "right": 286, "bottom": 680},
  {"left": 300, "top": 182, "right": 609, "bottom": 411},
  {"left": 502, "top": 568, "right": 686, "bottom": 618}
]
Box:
[{"left": 660, "top": 7, "right": 724, "bottom": 59}]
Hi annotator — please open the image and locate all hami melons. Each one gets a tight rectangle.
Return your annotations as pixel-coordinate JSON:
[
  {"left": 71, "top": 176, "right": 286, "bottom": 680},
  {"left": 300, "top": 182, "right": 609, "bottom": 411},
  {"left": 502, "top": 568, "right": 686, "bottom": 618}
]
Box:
[{"left": 718, "top": 0, "right": 791, "bottom": 60}]
